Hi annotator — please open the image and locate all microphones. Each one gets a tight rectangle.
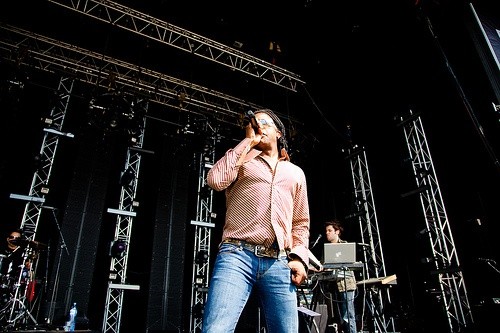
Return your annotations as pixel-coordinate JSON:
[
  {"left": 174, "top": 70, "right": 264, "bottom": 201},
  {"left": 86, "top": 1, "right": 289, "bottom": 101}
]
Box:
[
  {"left": 40, "top": 206, "right": 57, "bottom": 209},
  {"left": 478, "top": 258, "right": 494, "bottom": 262},
  {"left": 313, "top": 234, "right": 322, "bottom": 248},
  {"left": 247, "top": 110, "right": 261, "bottom": 135}
]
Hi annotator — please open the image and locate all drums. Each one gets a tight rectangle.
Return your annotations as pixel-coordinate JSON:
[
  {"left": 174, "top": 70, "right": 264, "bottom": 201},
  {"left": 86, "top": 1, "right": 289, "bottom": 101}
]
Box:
[
  {"left": 0, "top": 283, "right": 21, "bottom": 312},
  {"left": 15, "top": 263, "right": 35, "bottom": 283},
  {"left": 0, "top": 255, "right": 14, "bottom": 278},
  {"left": 21, "top": 249, "right": 41, "bottom": 260},
  {"left": 22, "top": 279, "right": 42, "bottom": 303}
]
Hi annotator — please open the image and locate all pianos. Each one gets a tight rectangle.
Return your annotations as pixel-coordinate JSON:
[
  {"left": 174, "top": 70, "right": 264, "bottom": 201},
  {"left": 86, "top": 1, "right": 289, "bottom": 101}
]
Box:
[{"left": 309, "top": 263, "right": 365, "bottom": 333}]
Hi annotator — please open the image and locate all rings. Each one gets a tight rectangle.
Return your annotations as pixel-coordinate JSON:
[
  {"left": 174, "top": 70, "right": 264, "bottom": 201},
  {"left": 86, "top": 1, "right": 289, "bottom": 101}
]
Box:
[
  {"left": 246, "top": 125, "right": 249, "bottom": 128},
  {"left": 304, "top": 279, "right": 306, "bottom": 282}
]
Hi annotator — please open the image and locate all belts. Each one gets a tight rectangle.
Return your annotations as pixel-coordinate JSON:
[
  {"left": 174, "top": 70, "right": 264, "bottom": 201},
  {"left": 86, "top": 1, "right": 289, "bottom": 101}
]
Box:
[{"left": 224, "top": 238, "right": 279, "bottom": 259}]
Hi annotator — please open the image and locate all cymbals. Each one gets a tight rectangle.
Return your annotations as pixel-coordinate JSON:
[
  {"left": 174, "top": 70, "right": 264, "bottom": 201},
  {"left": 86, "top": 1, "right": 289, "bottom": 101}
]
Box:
[{"left": 9, "top": 239, "right": 39, "bottom": 246}]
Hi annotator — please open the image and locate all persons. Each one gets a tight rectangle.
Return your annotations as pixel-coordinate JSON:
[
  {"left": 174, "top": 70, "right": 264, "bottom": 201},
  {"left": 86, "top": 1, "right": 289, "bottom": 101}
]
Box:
[
  {"left": 202, "top": 109, "right": 311, "bottom": 333},
  {"left": 0, "top": 229, "right": 35, "bottom": 333},
  {"left": 322, "top": 221, "right": 357, "bottom": 333}
]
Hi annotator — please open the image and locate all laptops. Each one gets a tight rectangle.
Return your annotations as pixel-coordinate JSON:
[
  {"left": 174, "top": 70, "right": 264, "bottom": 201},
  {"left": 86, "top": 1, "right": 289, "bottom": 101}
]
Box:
[{"left": 324, "top": 243, "right": 356, "bottom": 264}]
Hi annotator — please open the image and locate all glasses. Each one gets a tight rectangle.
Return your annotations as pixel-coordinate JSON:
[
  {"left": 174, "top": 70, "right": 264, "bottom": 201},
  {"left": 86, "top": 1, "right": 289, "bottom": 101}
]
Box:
[{"left": 259, "top": 119, "right": 280, "bottom": 133}]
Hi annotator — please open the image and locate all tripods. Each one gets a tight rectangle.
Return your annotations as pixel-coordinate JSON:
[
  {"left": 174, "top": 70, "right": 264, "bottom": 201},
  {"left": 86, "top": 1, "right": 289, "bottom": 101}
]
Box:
[{"left": 0, "top": 244, "right": 39, "bottom": 328}]
